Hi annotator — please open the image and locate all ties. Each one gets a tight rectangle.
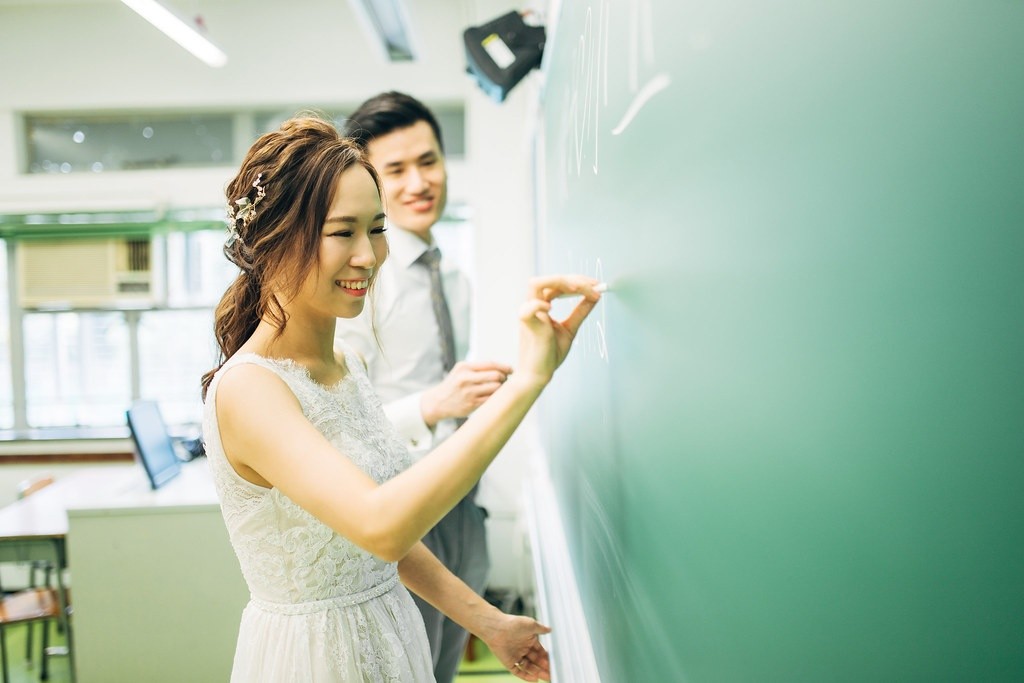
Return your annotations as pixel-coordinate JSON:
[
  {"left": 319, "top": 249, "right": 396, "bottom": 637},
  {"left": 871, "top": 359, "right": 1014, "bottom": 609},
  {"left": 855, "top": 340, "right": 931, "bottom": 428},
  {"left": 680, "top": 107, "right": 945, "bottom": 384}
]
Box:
[{"left": 415, "top": 246, "right": 458, "bottom": 372}]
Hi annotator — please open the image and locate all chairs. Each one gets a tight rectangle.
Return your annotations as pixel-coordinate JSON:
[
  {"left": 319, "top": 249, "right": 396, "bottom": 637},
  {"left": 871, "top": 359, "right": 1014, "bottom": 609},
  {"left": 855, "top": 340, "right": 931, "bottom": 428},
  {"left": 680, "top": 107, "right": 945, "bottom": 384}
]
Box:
[{"left": 0, "top": 535, "right": 76, "bottom": 683}]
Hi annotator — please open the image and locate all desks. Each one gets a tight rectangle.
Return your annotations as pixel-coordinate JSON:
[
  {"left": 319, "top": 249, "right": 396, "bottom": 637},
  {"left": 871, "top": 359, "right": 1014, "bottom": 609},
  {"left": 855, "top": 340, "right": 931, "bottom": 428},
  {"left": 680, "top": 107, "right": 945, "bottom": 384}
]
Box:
[{"left": 0, "top": 460, "right": 73, "bottom": 683}]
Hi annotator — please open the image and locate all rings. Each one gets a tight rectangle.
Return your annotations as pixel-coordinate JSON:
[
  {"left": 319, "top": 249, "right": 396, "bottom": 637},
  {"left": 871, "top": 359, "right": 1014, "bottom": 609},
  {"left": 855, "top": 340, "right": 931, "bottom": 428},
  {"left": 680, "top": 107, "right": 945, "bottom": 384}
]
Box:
[{"left": 515, "top": 657, "right": 527, "bottom": 669}]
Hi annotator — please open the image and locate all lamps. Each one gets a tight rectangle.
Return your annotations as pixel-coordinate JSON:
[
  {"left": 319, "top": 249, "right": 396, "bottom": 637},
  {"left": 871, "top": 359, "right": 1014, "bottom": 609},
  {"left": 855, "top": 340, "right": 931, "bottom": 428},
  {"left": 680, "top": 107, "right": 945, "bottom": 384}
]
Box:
[{"left": 464, "top": 8, "right": 548, "bottom": 103}]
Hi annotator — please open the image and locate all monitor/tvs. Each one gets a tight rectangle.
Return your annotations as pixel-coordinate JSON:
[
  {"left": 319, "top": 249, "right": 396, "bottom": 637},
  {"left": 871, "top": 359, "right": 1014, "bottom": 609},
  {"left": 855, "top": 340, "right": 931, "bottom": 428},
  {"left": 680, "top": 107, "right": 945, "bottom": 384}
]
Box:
[{"left": 126, "top": 400, "right": 180, "bottom": 489}]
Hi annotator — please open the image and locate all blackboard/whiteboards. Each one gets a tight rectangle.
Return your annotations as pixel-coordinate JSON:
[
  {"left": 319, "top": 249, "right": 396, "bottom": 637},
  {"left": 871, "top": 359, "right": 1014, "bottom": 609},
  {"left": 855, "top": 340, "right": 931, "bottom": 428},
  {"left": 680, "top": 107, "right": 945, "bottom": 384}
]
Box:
[{"left": 501, "top": 0, "right": 1024, "bottom": 683}]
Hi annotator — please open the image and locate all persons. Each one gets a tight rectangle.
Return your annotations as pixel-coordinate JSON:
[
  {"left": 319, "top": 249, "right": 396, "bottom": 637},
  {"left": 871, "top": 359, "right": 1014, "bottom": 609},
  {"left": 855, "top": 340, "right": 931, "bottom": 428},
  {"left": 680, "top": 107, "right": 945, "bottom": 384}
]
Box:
[
  {"left": 331, "top": 91, "right": 514, "bottom": 683},
  {"left": 193, "top": 119, "right": 599, "bottom": 683}
]
